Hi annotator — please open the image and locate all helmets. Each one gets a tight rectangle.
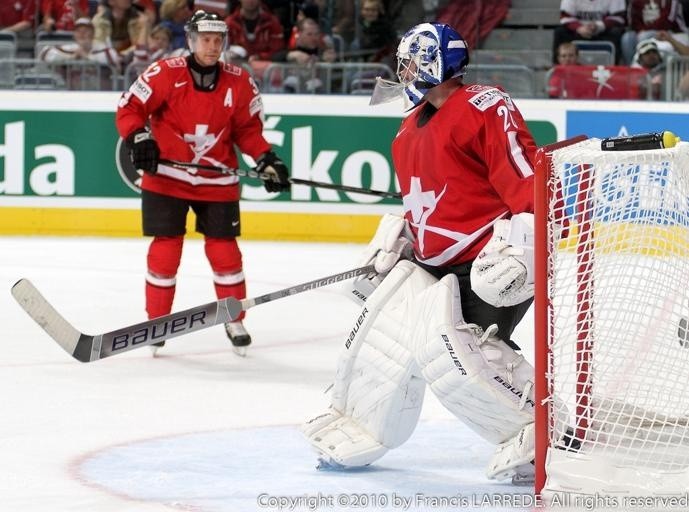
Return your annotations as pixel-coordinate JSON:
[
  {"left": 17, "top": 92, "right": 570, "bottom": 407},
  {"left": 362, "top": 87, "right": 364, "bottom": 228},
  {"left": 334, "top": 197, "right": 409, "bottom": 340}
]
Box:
[
  {"left": 396, "top": 21, "right": 470, "bottom": 101},
  {"left": 183, "top": 8, "right": 229, "bottom": 41}
]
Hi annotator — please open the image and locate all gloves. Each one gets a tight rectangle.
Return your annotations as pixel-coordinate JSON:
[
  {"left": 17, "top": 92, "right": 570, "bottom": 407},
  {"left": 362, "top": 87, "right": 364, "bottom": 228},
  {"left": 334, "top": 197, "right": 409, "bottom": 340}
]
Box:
[
  {"left": 253, "top": 152, "right": 291, "bottom": 192},
  {"left": 126, "top": 128, "right": 159, "bottom": 174},
  {"left": 470, "top": 212, "right": 562, "bottom": 308},
  {"left": 346, "top": 214, "right": 415, "bottom": 306}
]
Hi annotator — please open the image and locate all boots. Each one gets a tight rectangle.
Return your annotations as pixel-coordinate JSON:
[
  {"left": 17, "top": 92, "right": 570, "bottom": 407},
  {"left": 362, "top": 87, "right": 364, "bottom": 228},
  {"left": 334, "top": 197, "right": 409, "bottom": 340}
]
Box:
[
  {"left": 151, "top": 339, "right": 165, "bottom": 346},
  {"left": 224, "top": 320, "right": 251, "bottom": 346}
]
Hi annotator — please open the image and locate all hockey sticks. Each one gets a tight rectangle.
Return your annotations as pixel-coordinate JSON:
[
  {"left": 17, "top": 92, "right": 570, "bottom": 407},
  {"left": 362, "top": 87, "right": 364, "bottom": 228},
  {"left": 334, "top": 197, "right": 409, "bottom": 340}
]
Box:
[{"left": 11, "top": 265, "right": 377, "bottom": 363}]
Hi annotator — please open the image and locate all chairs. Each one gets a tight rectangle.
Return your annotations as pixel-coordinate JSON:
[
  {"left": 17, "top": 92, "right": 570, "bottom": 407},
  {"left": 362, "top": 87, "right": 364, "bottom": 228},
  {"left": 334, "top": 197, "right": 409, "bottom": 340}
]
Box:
[{"left": 0, "top": 1, "right": 689, "bottom": 102}]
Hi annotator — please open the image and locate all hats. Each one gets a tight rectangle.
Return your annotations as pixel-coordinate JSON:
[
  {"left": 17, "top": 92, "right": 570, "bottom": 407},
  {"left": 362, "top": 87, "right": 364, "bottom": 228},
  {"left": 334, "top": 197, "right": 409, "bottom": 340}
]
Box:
[
  {"left": 74, "top": 17, "right": 93, "bottom": 27},
  {"left": 637, "top": 40, "right": 657, "bottom": 54}
]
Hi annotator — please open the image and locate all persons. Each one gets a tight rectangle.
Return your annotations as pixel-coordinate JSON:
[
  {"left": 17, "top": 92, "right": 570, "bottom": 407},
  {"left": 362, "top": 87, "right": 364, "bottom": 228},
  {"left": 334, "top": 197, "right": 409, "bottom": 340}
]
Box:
[
  {"left": 0, "top": 0, "right": 689, "bottom": 99},
  {"left": 300, "top": 22, "right": 569, "bottom": 478},
  {"left": 114, "top": 9, "right": 290, "bottom": 346}
]
